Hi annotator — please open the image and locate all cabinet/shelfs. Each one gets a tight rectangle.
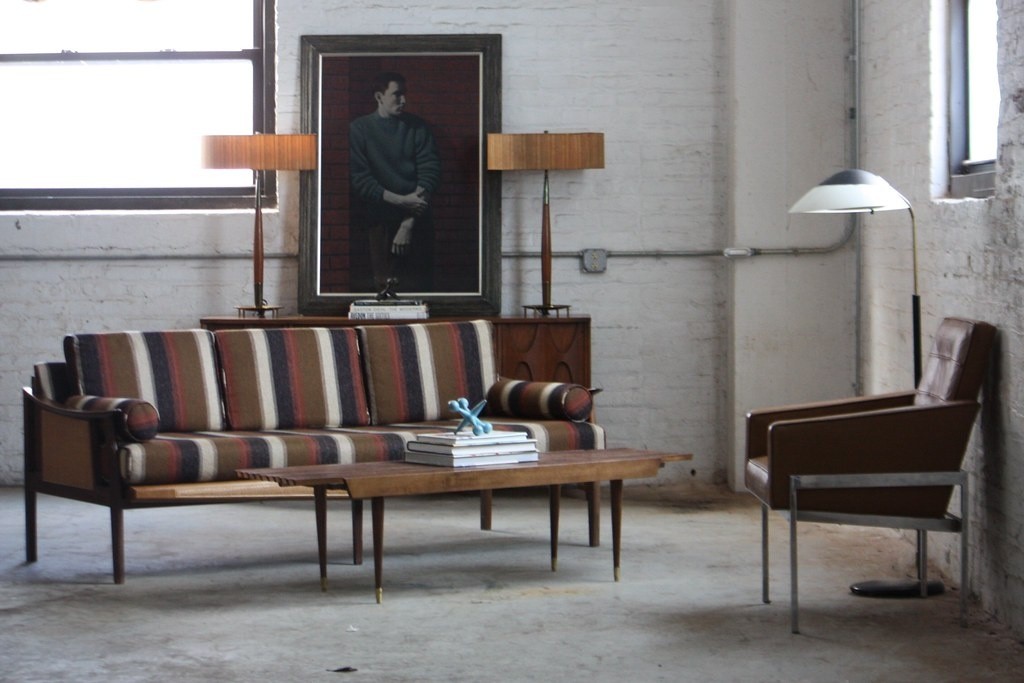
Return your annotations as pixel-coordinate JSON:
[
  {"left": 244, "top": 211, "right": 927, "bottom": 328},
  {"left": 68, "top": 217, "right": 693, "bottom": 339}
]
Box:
[{"left": 204, "top": 313, "right": 590, "bottom": 501}]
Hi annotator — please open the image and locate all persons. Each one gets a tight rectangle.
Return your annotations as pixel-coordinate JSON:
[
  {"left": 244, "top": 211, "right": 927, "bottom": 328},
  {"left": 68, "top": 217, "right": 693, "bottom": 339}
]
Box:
[{"left": 349, "top": 74, "right": 443, "bottom": 291}]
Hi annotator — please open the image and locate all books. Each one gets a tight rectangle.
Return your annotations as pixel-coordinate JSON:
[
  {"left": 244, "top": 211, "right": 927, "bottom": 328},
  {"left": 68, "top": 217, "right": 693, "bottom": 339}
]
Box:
[{"left": 400, "top": 429, "right": 538, "bottom": 468}]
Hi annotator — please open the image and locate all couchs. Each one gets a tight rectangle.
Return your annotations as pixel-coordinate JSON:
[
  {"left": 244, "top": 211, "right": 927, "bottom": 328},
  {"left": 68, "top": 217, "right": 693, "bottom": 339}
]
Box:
[{"left": 21, "top": 319, "right": 606, "bottom": 584}]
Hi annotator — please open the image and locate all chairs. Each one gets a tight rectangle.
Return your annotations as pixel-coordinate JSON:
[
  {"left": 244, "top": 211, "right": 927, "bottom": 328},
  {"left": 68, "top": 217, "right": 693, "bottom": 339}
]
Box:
[{"left": 744, "top": 317, "right": 996, "bottom": 635}]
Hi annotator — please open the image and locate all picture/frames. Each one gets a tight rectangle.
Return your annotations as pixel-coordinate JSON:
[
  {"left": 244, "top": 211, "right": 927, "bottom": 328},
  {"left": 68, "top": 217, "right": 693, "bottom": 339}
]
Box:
[{"left": 297, "top": 33, "right": 502, "bottom": 317}]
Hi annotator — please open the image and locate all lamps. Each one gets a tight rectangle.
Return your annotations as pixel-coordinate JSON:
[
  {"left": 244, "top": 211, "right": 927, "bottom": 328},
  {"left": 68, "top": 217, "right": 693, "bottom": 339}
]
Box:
[
  {"left": 200, "top": 132, "right": 317, "bottom": 316},
  {"left": 786, "top": 169, "right": 946, "bottom": 599},
  {"left": 488, "top": 131, "right": 604, "bottom": 314}
]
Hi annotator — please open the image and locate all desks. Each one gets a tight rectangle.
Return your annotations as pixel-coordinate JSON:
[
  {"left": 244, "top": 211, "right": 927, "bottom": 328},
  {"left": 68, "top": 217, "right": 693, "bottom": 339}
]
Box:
[{"left": 235, "top": 447, "right": 694, "bottom": 605}]
